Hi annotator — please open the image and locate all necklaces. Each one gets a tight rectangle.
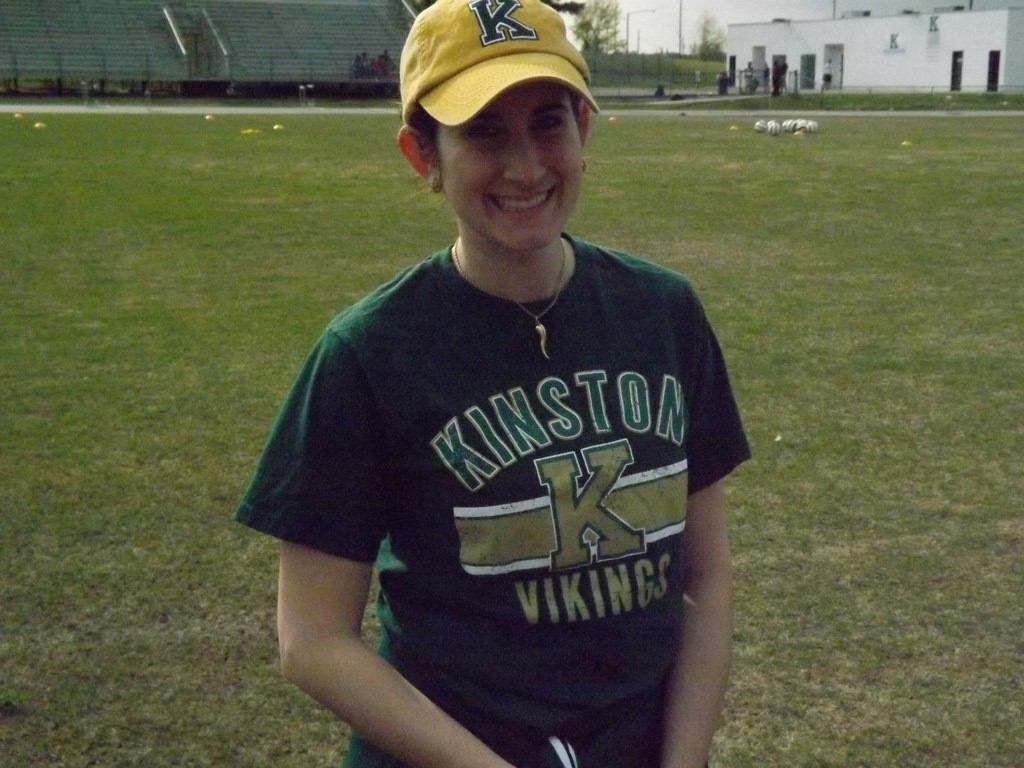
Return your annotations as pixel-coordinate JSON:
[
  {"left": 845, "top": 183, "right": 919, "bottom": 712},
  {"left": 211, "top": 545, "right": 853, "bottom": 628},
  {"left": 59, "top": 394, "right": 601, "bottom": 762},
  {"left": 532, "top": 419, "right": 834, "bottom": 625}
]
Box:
[{"left": 454, "top": 238, "right": 566, "bottom": 359}]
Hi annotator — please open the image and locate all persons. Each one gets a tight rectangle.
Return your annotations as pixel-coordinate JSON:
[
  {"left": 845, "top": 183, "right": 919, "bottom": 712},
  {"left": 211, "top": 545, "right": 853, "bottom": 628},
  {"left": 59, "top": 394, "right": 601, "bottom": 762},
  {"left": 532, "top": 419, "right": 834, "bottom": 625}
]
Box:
[
  {"left": 822, "top": 59, "right": 832, "bottom": 90},
  {"left": 351, "top": 48, "right": 391, "bottom": 80},
  {"left": 772, "top": 55, "right": 788, "bottom": 96},
  {"left": 762, "top": 62, "right": 770, "bottom": 94},
  {"left": 744, "top": 61, "right": 754, "bottom": 92},
  {"left": 236, "top": 0, "right": 751, "bottom": 768}
]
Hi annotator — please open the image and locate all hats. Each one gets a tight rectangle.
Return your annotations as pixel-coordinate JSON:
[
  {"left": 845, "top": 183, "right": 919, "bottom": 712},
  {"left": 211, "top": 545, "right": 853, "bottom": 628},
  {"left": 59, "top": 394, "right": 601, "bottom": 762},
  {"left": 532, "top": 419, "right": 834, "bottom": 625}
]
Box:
[{"left": 399, "top": 0, "right": 601, "bottom": 131}]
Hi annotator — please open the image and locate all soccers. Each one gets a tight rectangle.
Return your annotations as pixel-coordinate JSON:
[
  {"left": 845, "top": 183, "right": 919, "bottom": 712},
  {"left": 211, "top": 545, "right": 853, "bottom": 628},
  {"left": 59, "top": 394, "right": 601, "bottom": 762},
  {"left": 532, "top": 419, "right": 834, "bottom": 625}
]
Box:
[{"left": 754, "top": 118, "right": 819, "bottom": 136}]
